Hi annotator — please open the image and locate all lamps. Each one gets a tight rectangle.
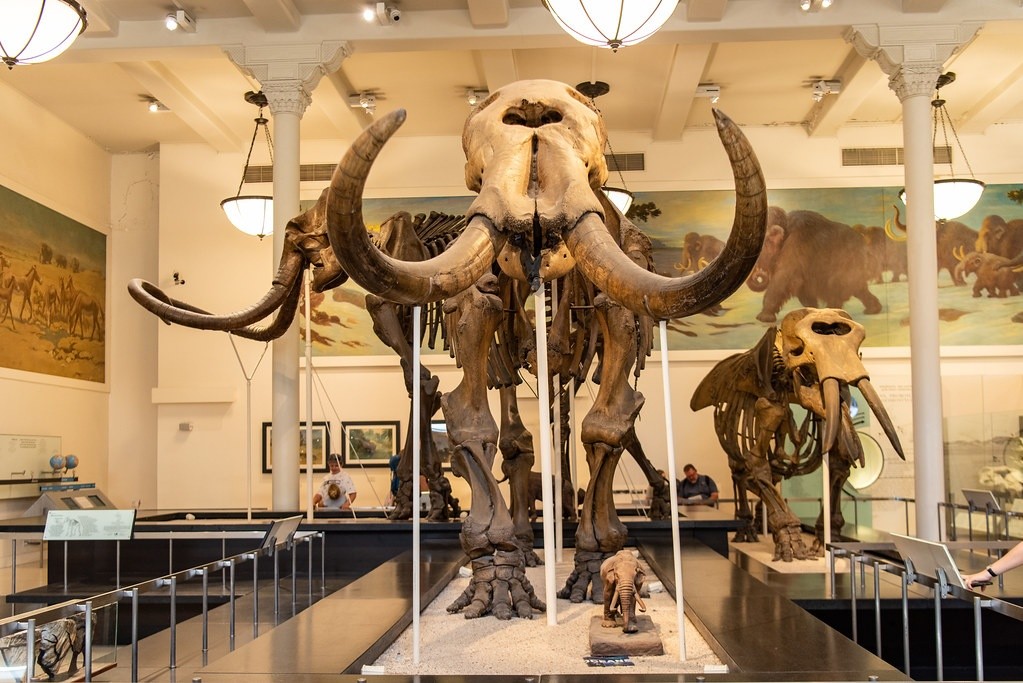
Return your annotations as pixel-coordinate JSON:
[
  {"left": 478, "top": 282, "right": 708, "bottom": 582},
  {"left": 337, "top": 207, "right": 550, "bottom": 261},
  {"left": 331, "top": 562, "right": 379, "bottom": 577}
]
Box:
[
  {"left": 573, "top": 80, "right": 637, "bottom": 216},
  {"left": 219, "top": 89, "right": 276, "bottom": 245},
  {"left": 897, "top": 72, "right": 989, "bottom": 226},
  {"left": 541, "top": 0, "right": 685, "bottom": 54},
  {"left": 0, "top": 0, "right": 91, "bottom": 71}
]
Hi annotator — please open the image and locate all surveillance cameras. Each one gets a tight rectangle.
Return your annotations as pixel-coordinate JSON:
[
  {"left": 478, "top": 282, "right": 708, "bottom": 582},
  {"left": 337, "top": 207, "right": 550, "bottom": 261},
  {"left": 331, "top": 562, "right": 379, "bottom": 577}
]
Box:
[{"left": 390, "top": 6, "right": 401, "bottom": 21}]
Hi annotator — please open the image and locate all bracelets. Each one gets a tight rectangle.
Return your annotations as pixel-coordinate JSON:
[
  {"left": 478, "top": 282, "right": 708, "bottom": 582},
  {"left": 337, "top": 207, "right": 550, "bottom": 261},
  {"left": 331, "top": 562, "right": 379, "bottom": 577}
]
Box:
[{"left": 986, "top": 566, "right": 998, "bottom": 578}]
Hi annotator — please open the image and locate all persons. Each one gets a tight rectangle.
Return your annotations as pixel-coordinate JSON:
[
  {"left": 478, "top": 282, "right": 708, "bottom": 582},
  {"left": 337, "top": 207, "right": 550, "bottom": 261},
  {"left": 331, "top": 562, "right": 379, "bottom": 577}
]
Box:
[
  {"left": 313, "top": 454, "right": 357, "bottom": 510},
  {"left": 645, "top": 469, "right": 669, "bottom": 506},
  {"left": 677, "top": 464, "right": 719, "bottom": 508},
  {"left": 960, "top": 542, "right": 1023, "bottom": 592}
]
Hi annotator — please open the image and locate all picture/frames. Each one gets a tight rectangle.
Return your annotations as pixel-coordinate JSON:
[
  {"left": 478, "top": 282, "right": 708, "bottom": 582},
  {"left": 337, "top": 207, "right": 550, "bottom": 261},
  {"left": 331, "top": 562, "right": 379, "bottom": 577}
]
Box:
[
  {"left": 292, "top": 171, "right": 1023, "bottom": 370},
  {"left": 260, "top": 420, "right": 332, "bottom": 475},
  {"left": 0, "top": 173, "right": 114, "bottom": 394},
  {"left": 429, "top": 419, "right": 452, "bottom": 472},
  {"left": 340, "top": 419, "right": 401, "bottom": 470}
]
